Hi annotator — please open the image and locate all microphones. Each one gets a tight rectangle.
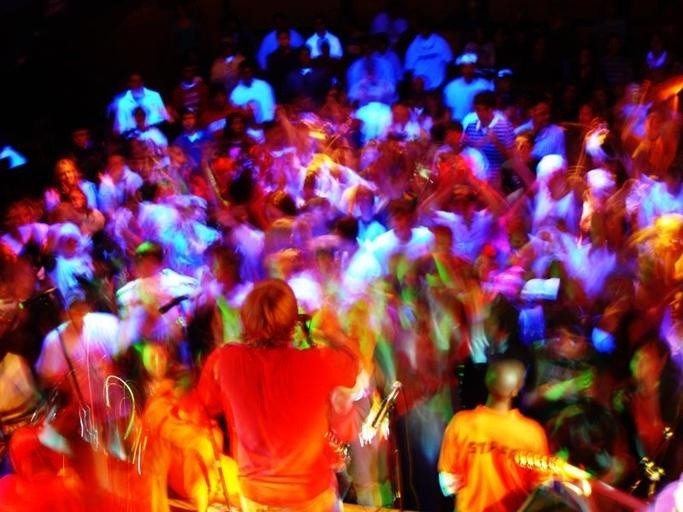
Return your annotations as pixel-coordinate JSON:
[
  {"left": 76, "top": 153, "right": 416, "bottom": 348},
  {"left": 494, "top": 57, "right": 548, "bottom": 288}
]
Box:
[
  {"left": 367, "top": 380, "right": 404, "bottom": 431},
  {"left": 17, "top": 286, "right": 63, "bottom": 313},
  {"left": 297, "top": 314, "right": 314, "bottom": 321},
  {"left": 158, "top": 293, "right": 189, "bottom": 314}
]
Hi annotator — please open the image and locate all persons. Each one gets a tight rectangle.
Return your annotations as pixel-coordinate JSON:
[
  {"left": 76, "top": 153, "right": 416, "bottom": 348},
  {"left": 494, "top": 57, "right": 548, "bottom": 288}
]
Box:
[{"left": 0, "top": 11, "right": 682, "bottom": 512}]
